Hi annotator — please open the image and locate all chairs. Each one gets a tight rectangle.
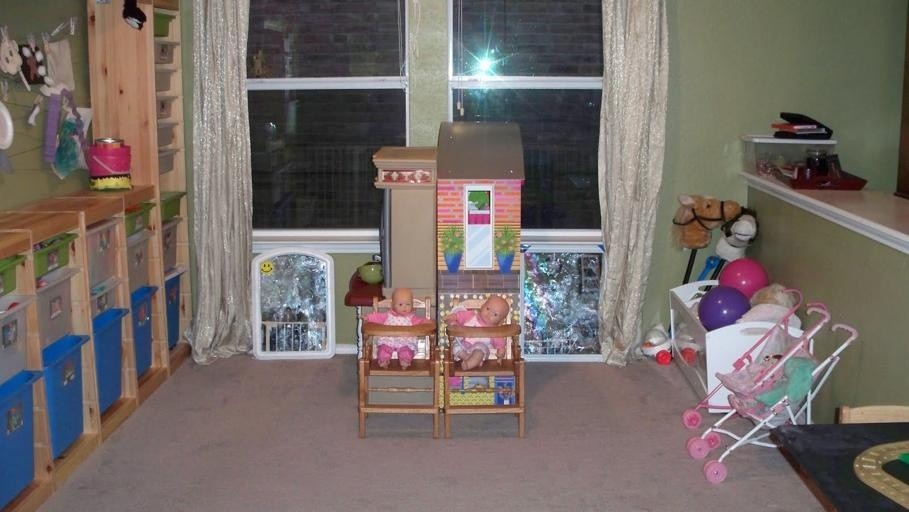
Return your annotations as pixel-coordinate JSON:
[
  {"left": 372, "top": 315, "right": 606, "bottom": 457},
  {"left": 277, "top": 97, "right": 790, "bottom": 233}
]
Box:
[
  {"left": 358, "top": 293, "right": 440, "bottom": 439},
  {"left": 444, "top": 298, "right": 525, "bottom": 438},
  {"left": 835, "top": 403, "right": 909, "bottom": 423}
]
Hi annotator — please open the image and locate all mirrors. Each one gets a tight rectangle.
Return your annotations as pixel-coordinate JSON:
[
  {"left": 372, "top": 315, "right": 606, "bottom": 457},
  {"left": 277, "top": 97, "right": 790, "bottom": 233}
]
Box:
[
  {"left": 521, "top": 244, "right": 607, "bottom": 363},
  {"left": 252, "top": 247, "right": 336, "bottom": 361}
]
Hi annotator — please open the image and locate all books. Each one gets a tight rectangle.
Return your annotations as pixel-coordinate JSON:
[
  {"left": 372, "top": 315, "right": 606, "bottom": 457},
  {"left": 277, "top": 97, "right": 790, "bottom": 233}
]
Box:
[{"left": 770, "top": 122, "right": 827, "bottom": 136}]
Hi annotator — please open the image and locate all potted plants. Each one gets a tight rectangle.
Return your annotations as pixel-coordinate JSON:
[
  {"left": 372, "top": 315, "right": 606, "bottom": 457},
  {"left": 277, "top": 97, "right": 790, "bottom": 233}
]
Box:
[
  {"left": 441, "top": 226, "right": 465, "bottom": 272},
  {"left": 495, "top": 226, "right": 517, "bottom": 273}
]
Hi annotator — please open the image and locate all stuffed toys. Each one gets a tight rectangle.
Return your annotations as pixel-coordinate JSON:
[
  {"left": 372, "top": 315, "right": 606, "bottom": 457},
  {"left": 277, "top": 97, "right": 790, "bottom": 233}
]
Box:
[
  {"left": 739, "top": 354, "right": 782, "bottom": 390},
  {"left": 671, "top": 195, "right": 740, "bottom": 248},
  {"left": 716, "top": 208, "right": 756, "bottom": 266},
  {"left": 744, "top": 356, "right": 815, "bottom": 416}
]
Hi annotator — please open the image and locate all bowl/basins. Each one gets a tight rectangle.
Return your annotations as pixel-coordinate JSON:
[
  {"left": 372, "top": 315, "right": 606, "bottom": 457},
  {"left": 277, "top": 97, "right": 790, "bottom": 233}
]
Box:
[{"left": 358, "top": 265, "right": 383, "bottom": 286}]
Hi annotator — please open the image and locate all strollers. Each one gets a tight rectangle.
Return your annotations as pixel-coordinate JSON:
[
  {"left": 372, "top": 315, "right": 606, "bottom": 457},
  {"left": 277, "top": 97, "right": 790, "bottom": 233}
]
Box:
[{"left": 681, "top": 289, "right": 858, "bottom": 484}]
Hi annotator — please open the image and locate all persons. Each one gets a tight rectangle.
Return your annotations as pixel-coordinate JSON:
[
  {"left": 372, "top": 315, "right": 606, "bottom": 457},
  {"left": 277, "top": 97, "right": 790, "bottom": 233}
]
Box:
[
  {"left": 446, "top": 296, "right": 508, "bottom": 370},
  {"left": 365, "top": 289, "right": 430, "bottom": 368}
]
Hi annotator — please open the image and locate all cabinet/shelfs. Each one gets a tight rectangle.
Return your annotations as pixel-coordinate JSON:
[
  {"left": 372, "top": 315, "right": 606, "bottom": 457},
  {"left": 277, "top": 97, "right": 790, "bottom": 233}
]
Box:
[
  {"left": 0, "top": 190, "right": 174, "bottom": 477},
  {"left": 87, "top": 0, "right": 194, "bottom": 368}
]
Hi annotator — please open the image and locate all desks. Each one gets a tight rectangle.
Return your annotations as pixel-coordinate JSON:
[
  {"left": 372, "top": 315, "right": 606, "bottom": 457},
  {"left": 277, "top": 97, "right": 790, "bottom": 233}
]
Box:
[{"left": 772, "top": 423, "right": 909, "bottom": 512}]
